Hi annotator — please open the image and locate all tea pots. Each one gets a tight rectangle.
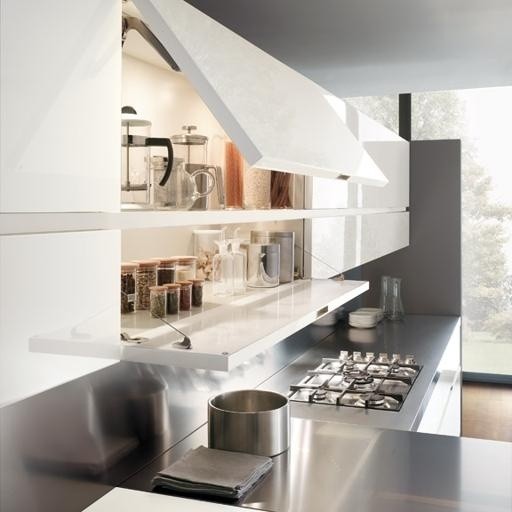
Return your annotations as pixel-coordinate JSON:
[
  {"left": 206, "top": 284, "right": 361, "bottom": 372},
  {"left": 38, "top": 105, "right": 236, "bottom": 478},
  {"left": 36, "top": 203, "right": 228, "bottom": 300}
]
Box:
[
  {"left": 170, "top": 125, "right": 218, "bottom": 210},
  {"left": 120, "top": 105, "right": 173, "bottom": 210},
  {"left": 153, "top": 156, "right": 215, "bottom": 210}
]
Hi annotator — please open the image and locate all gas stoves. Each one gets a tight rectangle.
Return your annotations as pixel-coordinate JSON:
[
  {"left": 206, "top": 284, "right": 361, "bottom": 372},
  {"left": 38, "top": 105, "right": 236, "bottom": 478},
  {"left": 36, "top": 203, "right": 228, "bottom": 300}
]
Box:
[{"left": 288, "top": 350, "right": 424, "bottom": 412}]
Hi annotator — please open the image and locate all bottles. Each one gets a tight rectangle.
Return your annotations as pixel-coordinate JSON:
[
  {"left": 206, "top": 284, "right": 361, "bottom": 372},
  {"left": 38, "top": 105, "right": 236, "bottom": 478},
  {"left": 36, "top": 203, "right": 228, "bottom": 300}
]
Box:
[
  {"left": 348, "top": 307, "right": 383, "bottom": 329},
  {"left": 229, "top": 239, "right": 246, "bottom": 295},
  {"left": 213, "top": 240, "right": 233, "bottom": 297},
  {"left": 224, "top": 140, "right": 244, "bottom": 210},
  {"left": 121, "top": 256, "right": 203, "bottom": 320},
  {"left": 192, "top": 230, "right": 224, "bottom": 283},
  {"left": 387, "top": 278, "right": 405, "bottom": 321},
  {"left": 380, "top": 276, "right": 391, "bottom": 318}
]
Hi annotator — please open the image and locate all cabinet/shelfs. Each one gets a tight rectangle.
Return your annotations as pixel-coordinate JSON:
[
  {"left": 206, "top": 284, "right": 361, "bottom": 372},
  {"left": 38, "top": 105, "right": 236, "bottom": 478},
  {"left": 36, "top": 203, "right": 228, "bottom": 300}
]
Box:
[
  {"left": 303, "top": 87, "right": 411, "bottom": 277},
  {"left": 413, "top": 317, "right": 463, "bottom": 438},
  {"left": 1, "top": 0, "right": 389, "bottom": 407}
]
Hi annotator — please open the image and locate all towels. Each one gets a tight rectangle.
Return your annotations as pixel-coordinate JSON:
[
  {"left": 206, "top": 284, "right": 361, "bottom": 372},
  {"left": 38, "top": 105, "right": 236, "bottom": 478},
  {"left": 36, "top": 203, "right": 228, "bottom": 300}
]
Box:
[{"left": 151, "top": 444, "right": 276, "bottom": 501}]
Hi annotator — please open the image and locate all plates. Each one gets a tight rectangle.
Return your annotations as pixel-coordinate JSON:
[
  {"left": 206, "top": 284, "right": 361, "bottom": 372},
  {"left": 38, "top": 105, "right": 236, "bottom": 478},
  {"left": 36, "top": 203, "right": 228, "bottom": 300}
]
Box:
[
  {"left": 312, "top": 305, "right": 345, "bottom": 326},
  {"left": 348, "top": 328, "right": 380, "bottom": 344}
]
